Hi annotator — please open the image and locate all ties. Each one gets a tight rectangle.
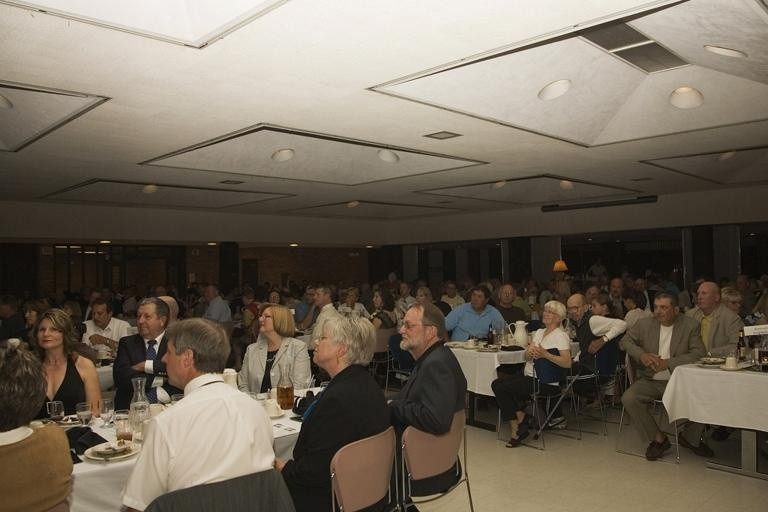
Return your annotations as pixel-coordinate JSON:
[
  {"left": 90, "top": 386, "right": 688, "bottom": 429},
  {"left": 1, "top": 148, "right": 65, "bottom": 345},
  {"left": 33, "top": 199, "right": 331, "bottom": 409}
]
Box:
[{"left": 141, "top": 333, "right": 157, "bottom": 404}]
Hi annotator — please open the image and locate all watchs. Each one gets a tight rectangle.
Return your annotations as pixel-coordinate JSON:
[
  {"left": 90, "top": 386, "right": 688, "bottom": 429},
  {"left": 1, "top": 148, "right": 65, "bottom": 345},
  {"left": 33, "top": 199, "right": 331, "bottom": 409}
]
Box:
[
  {"left": 105, "top": 337, "right": 110, "bottom": 346},
  {"left": 602, "top": 336, "right": 609, "bottom": 344}
]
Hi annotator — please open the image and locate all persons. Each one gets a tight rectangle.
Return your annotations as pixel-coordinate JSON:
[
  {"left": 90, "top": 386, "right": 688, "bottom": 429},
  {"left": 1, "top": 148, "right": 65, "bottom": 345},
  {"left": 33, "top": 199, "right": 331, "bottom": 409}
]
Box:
[
  {"left": 387, "top": 302, "right": 466, "bottom": 511},
  {"left": 309, "top": 287, "right": 345, "bottom": 353},
  {"left": 1, "top": 295, "right": 28, "bottom": 342},
  {"left": 0, "top": 343, "right": 75, "bottom": 512},
  {"left": 441, "top": 281, "right": 465, "bottom": 309},
  {"left": 444, "top": 285, "right": 508, "bottom": 342},
  {"left": 384, "top": 268, "right": 522, "bottom": 289},
  {"left": 100, "top": 285, "right": 156, "bottom": 302},
  {"left": 35, "top": 308, "right": 104, "bottom": 420},
  {"left": 112, "top": 297, "right": 184, "bottom": 411},
  {"left": 237, "top": 307, "right": 313, "bottom": 394},
  {"left": 620, "top": 292, "right": 716, "bottom": 461},
  {"left": 416, "top": 288, "right": 435, "bottom": 305},
  {"left": 519, "top": 278, "right": 563, "bottom": 299},
  {"left": 18, "top": 285, "right": 101, "bottom": 310},
  {"left": 493, "top": 286, "right": 527, "bottom": 334},
  {"left": 492, "top": 301, "right": 572, "bottom": 448},
  {"left": 393, "top": 281, "right": 415, "bottom": 319},
  {"left": 720, "top": 273, "right": 768, "bottom": 324},
  {"left": 372, "top": 288, "right": 398, "bottom": 327},
  {"left": 62, "top": 300, "right": 82, "bottom": 327},
  {"left": 545, "top": 294, "right": 627, "bottom": 430},
  {"left": 581, "top": 263, "right": 684, "bottom": 311},
  {"left": 21, "top": 300, "right": 49, "bottom": 349},
  {"left": 202, "top": 284, "right": 231, "bottom": 322},
  {"left": 234, "top": 280, "right": 317, "bottom": 328},
  {"left": 154, "top": 280, "right": 207, "bottom": 307},
  {"left": 275, "top": 314, "right": 395, "bottom": 512},
  {"left": 684, "top": 282, "right": 742, "bottom": 357},
  {"left": 80, "top": 300, "right": 130, "bottom": 364},
  {"left": 338, "top": 287, "right": 370, "bottom": 317},
  {"left": 123, "top": 319, "right": 276, "bottom": 512}
]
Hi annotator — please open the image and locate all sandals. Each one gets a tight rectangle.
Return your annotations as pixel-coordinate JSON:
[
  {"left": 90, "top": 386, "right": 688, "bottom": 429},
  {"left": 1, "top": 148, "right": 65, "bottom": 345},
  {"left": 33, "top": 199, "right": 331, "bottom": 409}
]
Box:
[
  {"left": 506, "top": 428, "right": 531, "bottom": 448},
  {"left": 521, "top": 413, "right": 537, "bottom": 434}
]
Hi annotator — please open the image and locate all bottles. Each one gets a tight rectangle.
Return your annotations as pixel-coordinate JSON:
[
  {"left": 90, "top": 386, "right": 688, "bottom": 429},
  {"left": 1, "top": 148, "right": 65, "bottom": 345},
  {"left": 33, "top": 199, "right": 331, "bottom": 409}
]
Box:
[
  {"left": 131, "top": 375, "right": 150, "bottom": 426},
  {"left": 737, "top": 328, "right": 749, "bottom": 361},
  {"left": 278, "top": 362, "right": 292, "bottom": 408},
  {"left": 486, "top": 323, "right": 497, "bottom": 348}
]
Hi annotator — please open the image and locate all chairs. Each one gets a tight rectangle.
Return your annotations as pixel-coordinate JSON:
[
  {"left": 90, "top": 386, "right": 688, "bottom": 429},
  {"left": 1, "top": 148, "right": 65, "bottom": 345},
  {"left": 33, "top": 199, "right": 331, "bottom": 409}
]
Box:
[
  {"left": 615, "top": 350, "right": 682, "bottom": 465},
  {"left": 328, "top": 426, "right": 400, "bottom": 512},
  {"left": 368, "top": 326, "right": 397, "bottom": 379},
  {"left": 523, "top": 348, "right": 584, "bottom": 450},
  {"left": 385, "top": 333, "right": 415, "bottom": 394},
  {"left": 402, "top": 408, "right": 475, "bottom": 512},
  {"left": 545, "top": 336, "right": 624, "bottom": 441}
]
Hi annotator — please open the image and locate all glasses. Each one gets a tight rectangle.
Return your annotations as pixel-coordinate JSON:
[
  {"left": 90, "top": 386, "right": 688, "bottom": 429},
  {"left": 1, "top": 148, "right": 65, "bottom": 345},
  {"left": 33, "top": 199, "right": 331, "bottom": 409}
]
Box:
[
  {"left": 728, "top": 300, "right": 743, "bottom": 306},
  {"left": 566, "top": 304, "right": 582, "bottom": 314},
  {"left": 542, "top": 308, "right": 560, "bottom": 316},
  {"left": 397, "top": 320, "right": 434, "bottom": 330}
]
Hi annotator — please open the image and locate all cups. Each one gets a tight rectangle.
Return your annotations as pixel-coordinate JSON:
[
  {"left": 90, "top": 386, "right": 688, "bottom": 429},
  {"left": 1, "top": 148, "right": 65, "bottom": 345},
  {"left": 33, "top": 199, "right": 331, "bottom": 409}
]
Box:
[
  {"left": 304, "top": 379, "right": 315, "bottom": 396},
  {"left": 172, "top": 394, "right": 184, "bottom": 406},
  {"left": 467, "top": 339, "right": 473, "bottom": 347},
  {"left": 725, "top": 358, "right": 735, "bottom": 368},
  {"left": 74, "top": 402, "right": 92, "bottom": 429},
  {"left": 113, "top": 410, "right": 132, "bottom": 443},
  {"left": 46, "top": 401, "right": 64, "bottom": 424},
  {"left": 264, "top": 398, "right": 281, "bottom": 413},
  {"left": 320, "top": 381, "right": 330, "bottom": 391},
  {"left": 98, "top": 398, "right": 114, "bottom": 430}
]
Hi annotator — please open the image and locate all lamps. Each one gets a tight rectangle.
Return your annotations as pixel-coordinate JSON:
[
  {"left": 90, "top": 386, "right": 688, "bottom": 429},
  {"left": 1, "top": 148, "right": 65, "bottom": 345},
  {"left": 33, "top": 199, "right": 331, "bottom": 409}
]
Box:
[{"left": 553, "top": 235, "right": 567, "bottom": 272}]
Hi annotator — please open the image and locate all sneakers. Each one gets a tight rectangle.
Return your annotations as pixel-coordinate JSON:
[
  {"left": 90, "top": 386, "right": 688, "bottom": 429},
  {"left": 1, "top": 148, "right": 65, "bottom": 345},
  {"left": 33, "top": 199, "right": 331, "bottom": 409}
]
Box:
[{"left": 544, "top": 416, "right": 568, "bottom": 431}]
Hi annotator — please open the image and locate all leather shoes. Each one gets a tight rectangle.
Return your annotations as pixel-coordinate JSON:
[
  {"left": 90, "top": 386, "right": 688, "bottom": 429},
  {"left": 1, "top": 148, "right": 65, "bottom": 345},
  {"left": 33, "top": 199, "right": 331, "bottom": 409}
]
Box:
[
  {"left": 645, "top": 435, "right": 671, "bottom": 460},
  {"left": 678, "top": 431, "right": 714, "bottom": 458}
]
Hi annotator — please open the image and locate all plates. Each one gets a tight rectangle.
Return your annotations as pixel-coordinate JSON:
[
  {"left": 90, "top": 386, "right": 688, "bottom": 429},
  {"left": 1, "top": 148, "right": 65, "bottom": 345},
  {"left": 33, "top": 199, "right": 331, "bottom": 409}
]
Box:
[
  {"left": 461, "top": 345, "right": 475, "bottom": 350},
  {"left": 91, "top": 440, "right": 131, "bottom": 454},
  {"left": 445, "top": 342, "right": 462, "bottom": 348},
  {"left": 62, "top": 414, "right": 79, "bottom": 425},
  {"left": 700, "top": 357, "right": 724, "bottom": 364},
  {"left": 264, "top": 411, "right": 284, "bottom": 418},
  {"left": 83, "top": 442, "right": 141, "bottom": 461},
  {"left": 718, "top": 364, "right": 742, "bottom": 371}
]
